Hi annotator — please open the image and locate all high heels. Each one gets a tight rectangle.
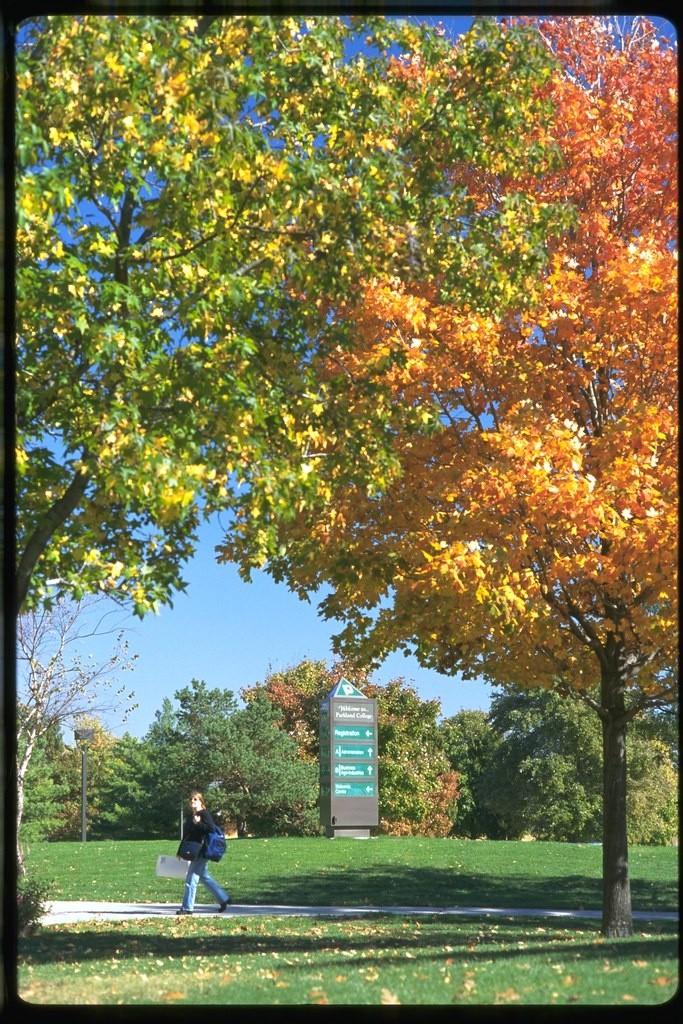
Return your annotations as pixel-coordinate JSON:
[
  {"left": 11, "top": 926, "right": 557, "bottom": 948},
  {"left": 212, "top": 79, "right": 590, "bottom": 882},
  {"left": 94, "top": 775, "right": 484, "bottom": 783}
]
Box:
[
  {"left": 218, "top": 897, "right": 231, "bottom": 912},
  {"left": 176, "top": 909, "right": 193, "bottom": 915}
]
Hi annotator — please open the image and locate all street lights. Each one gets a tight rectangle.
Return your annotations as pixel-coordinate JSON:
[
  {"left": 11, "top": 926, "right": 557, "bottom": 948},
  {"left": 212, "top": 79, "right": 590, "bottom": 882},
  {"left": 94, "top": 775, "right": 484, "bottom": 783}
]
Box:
[{"left": 74, "top": 729, "right": 94, "bottom": 843}]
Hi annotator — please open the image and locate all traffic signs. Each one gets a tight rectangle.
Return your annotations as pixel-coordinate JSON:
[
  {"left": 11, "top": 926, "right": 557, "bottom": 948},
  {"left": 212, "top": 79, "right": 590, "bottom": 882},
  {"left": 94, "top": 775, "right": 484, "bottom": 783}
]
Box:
[{"left": 332, "top": 726, "right": 375, "bottom": 796}]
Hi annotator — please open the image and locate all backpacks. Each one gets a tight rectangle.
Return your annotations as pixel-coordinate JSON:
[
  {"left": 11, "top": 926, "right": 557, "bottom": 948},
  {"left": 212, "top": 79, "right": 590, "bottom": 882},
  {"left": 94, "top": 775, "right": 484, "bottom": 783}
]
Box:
[{"left": 204, "top": 825, "right": 227, "bottom": 862}]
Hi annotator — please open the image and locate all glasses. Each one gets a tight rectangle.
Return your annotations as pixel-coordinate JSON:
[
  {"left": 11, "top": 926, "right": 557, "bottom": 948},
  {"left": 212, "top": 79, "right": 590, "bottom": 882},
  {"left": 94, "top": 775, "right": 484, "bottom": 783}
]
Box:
[{"left": 189, "top": 798, "right": 197, "bottom": 801}]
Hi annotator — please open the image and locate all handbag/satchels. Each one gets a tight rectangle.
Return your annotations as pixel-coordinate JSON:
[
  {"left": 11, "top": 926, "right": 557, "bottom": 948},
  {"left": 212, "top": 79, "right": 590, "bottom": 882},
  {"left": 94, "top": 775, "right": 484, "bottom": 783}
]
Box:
[
  {"left": 177, "top": 840, "right": 203, "bottom": 862},
  {"left": 155, "top": 855, "right": 191, "bottom": 880}
]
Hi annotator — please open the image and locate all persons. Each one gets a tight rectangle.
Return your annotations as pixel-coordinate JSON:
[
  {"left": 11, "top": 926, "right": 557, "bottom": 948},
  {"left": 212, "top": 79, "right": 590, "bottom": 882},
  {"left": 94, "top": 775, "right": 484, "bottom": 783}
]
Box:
[{"left": 177, "top": 792, "right": 233, "bottom": 915}]
